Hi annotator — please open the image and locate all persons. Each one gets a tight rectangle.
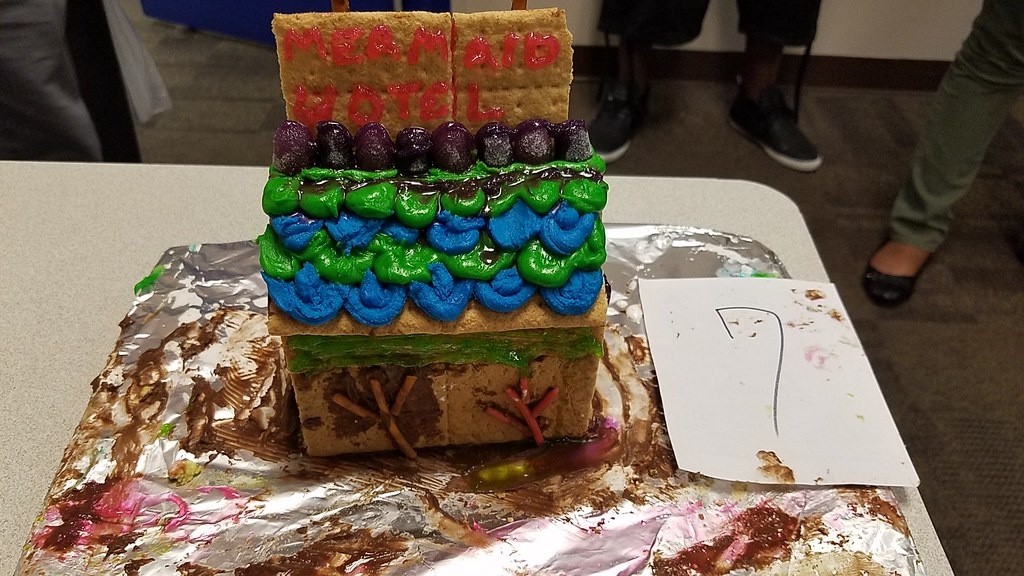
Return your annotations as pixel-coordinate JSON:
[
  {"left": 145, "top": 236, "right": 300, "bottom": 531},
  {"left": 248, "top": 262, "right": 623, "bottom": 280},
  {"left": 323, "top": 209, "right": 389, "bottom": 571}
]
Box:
[
  {"left": 580, "top": 0, "right": 824, "bottom": 171},
  {"left": 862, "top": 0, "right": 1023, "bottom": 307}
]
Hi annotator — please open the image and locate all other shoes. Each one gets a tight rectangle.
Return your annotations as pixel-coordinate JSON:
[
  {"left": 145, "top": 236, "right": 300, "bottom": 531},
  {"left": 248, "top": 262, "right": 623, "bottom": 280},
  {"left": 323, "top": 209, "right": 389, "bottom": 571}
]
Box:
[{"left": 862, "top": 235, "right": 938, "bottom": 307}]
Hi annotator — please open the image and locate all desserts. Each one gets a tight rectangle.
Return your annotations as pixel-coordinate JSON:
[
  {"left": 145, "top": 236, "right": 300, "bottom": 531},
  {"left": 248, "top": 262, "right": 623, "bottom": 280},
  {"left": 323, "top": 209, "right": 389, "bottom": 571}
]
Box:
[{"left": 248, "top": 11, "right": 608, "bottom": 458}]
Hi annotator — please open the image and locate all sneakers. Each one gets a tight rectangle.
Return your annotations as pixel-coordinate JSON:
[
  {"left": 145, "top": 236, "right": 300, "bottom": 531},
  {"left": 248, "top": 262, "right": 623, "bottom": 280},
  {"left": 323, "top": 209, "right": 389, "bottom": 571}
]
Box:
[
  {"left": 728, "top": 90, "right": 823, "bottom": 173},
  {"left": 585, "top": 92, "right": 650, "bottom": 162}
]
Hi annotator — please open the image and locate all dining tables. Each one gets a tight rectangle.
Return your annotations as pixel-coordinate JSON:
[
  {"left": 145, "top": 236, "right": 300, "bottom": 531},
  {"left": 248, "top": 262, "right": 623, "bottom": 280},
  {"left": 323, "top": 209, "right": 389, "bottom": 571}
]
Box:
[{"left": 1, "top": 156, "right": 954, "bottom": 574}]
